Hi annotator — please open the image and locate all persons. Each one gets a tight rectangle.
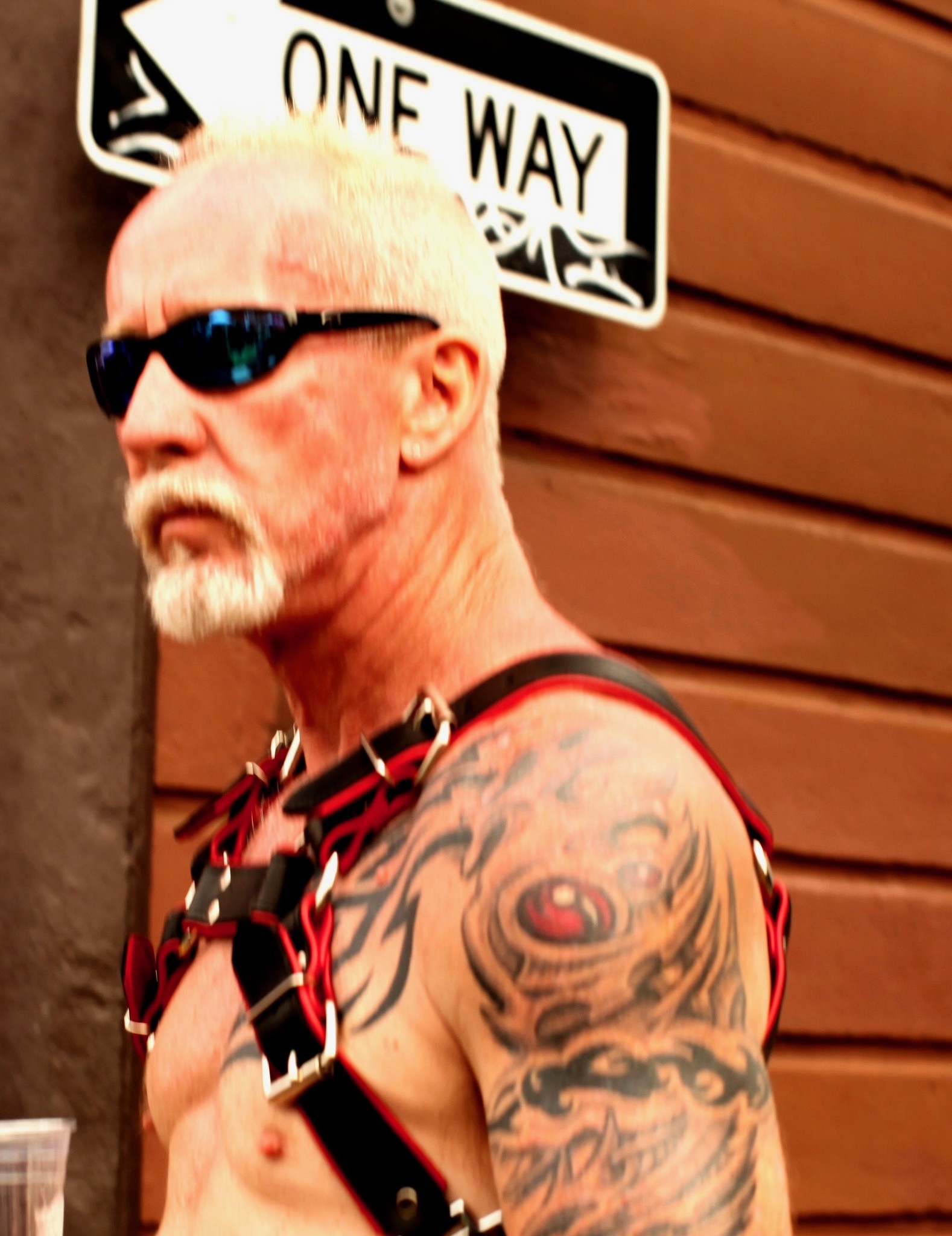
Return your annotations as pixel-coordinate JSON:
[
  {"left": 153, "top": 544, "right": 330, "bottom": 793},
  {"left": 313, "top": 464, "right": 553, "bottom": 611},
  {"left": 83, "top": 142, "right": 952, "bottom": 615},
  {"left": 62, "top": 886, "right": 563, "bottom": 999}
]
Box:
[{"left": 84, "top": 121, "right": 789, "bottom": 1236}]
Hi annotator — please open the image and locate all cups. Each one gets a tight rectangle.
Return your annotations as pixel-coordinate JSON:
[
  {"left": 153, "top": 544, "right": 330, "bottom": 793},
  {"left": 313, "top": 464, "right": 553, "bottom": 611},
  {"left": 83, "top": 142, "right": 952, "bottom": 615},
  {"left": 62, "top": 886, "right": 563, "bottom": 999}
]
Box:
[{"left": 0, "top": 1119, "right": 75, "bottom": 1236}]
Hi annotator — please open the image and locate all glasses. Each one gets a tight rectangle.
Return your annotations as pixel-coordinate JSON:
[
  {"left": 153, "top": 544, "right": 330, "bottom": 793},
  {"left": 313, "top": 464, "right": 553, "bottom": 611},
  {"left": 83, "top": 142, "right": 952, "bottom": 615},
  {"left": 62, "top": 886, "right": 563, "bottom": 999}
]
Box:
[{"left": 86, "top": 305, "right": 439, "bottom": 420}]
